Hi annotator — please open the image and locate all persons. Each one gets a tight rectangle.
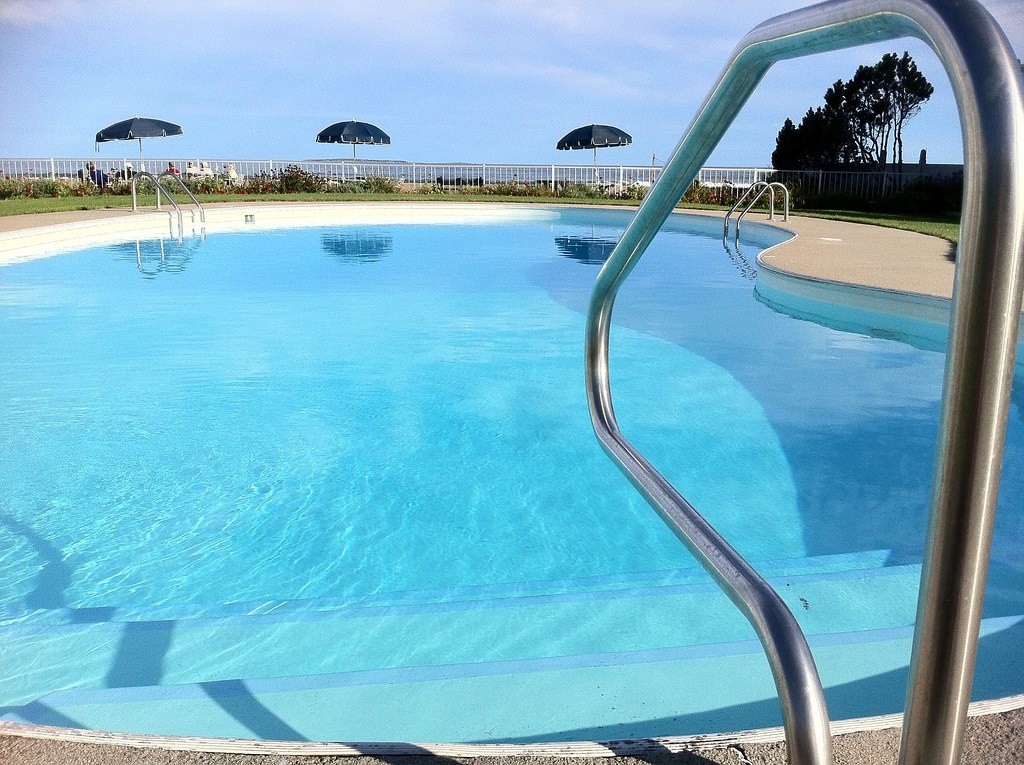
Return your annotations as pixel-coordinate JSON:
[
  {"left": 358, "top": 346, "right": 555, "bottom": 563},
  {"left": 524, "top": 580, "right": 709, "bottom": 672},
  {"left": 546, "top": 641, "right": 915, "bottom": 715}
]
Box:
[
  {"left": 606, "top": 184, "right": 615, "bottom": 197},
  {"left": 116, "top": 163, "right": 138, "bottom": 180},
  {"left": 166, "top": 162, "right": 180, "bottom": 173},
  {"left": 85, "top": 162, "right": 114, "bottom": 186},
  {"left": 222, "top": 165, "right": 238, "bottom": 177},
  {"left": 187, "top": 162, "right": 197, "bottom": 172}
]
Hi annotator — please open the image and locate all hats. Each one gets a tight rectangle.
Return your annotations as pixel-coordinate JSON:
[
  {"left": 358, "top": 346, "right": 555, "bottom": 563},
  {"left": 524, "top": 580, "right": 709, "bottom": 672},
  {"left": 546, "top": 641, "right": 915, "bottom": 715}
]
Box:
[{"left": 169, "top": 162, "right": 174, "bottom": 167}]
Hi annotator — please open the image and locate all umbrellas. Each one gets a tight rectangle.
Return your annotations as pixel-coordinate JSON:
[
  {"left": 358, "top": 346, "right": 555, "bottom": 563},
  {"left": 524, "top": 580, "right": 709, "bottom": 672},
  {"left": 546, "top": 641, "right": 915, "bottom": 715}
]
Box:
[
  {"left": 315, "top": 120, "right": 390, "bottom": 179},
  {"left": 96, "top": 117, "right": 184, "bottom": 179},
  {"left": 556, "top": 124, "right": 632, "bottom": 177}
]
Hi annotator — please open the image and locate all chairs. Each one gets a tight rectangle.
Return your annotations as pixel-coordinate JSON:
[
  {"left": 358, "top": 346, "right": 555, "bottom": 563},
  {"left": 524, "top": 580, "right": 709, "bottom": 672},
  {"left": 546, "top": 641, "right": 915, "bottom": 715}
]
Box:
[{"left": 84, "top": 166, "right": 246, "bottom": 188}]
